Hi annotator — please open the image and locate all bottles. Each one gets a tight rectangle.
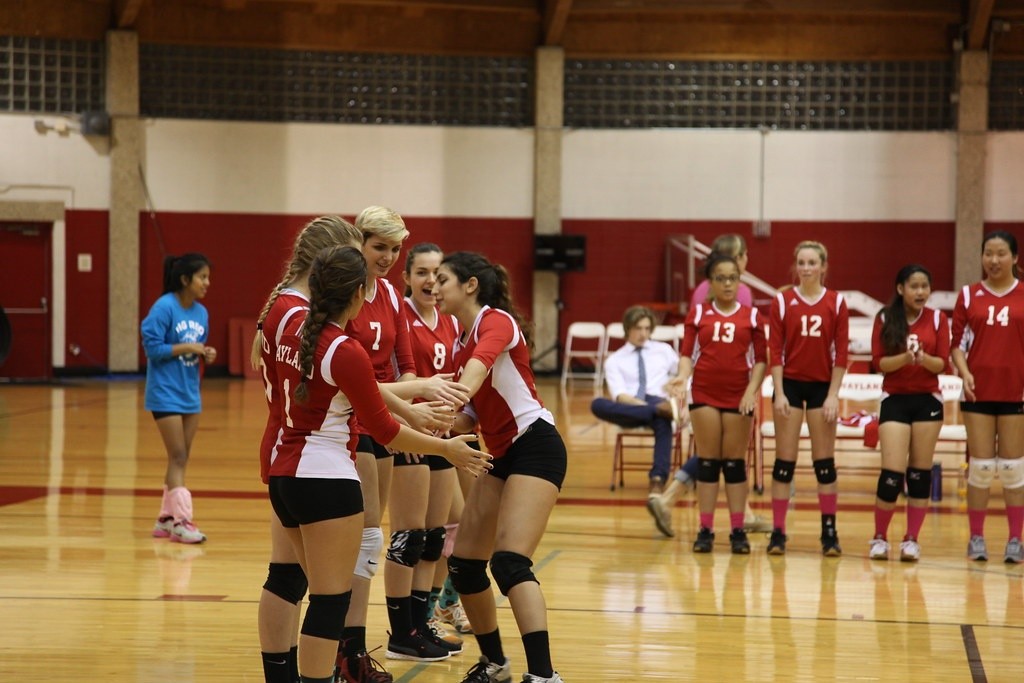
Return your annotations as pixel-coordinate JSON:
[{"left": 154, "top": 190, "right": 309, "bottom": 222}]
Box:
[{"left": 931, "top": 464, "right": 943, "bottom": 501}]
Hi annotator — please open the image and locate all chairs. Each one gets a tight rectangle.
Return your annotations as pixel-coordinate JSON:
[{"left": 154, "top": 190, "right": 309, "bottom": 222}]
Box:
[{"left": 561, "top": 318, "right": 969, "bottom": 493}]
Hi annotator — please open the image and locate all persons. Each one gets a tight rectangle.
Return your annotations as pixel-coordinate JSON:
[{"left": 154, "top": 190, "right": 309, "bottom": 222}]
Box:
[
  {"left": 142, "top": 253, "right": 217, "bottom": 542},
  {"left": 768, "top": 242, "right": 848, "bottom": 557},
  {"left": 868, "top": 264, "right": 949, "bottom": 561},
  {"left": 268, "top": 244, "right": 494, "bottom": 683},
  {"left": 648, "top": 233, "right": 770, "bottom": 537},
  {"left": 251, "top": 204, "right": 567, "bottom": 682},
  {"left": 591, "top": 305, "right": 679, "bottom": 502},
  {"left": 669, "top": 256, "right": 768, "bottom": 553},
  {"left": 949, "top": 230, "right": 1024, "bottom": 564}
]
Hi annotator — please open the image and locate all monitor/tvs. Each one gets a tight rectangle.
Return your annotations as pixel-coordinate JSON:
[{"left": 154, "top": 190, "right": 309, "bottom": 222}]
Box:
[{"left": 533, "top": 235, "right": 587, "bottom": 270}]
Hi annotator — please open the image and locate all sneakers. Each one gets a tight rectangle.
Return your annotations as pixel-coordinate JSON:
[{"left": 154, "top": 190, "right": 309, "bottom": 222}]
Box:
[
  {"left": 1004, "top": 538, "right": 1024, "bottom": 562},
  {"left": 968, "top": 537, "right": 988, "bottom": 561},
  {"left": 769, "top": 527, "right": 786, "bottom": 554},
  {"left": 170, "top": 519, "right": 205, "bottom": 542},
  {"left": 900, "top": 540, "right": 918, "bottom": 559},
  {"left": 434, "top": 603, "right": 472, "bottom": 632},
  {"left": 729, "top": 527, "right": 749, "bottom": 554},
  {"left": 385, "top": 630, "right": 450, "bottom": 661},
  {"left": 648, "top": 496, "right": 675, "bottom": 536},
  {"left": 151, "top": 518, "right": 174, "bottom": 537},
  {"left": 870, "top": 540, "right": 888, "bottom": 559},
  {"left": 339, "top": 644, "right": 393, "bottom": 683},
  {"left": 419, "top": 626, "right": 463, "bottom": 653},
  {"left": 459, "top": 656, "right": 511, "bottom": 683},
  {"left": 693, "top": 528, "right": 715, "bottom": 552},
  {"left": 520, "top": 671, "right": 563, "bottom": 683},
  {"left": 428, "top": 619, "right": 463, "bottom": 643},
  {"left": 820, "top": 529, "right": 841, "bottom": 554}
]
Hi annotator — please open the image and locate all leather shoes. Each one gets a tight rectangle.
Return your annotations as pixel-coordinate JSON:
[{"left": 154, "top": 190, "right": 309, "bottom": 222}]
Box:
[{"left": 634, "top": 347, "right": 647, "bottom": 399}]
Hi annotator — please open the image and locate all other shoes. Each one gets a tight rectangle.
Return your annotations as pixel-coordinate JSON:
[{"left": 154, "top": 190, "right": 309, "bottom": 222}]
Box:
[{"left": 658, "top": 397, "right": 679, "bottom": 425}]
